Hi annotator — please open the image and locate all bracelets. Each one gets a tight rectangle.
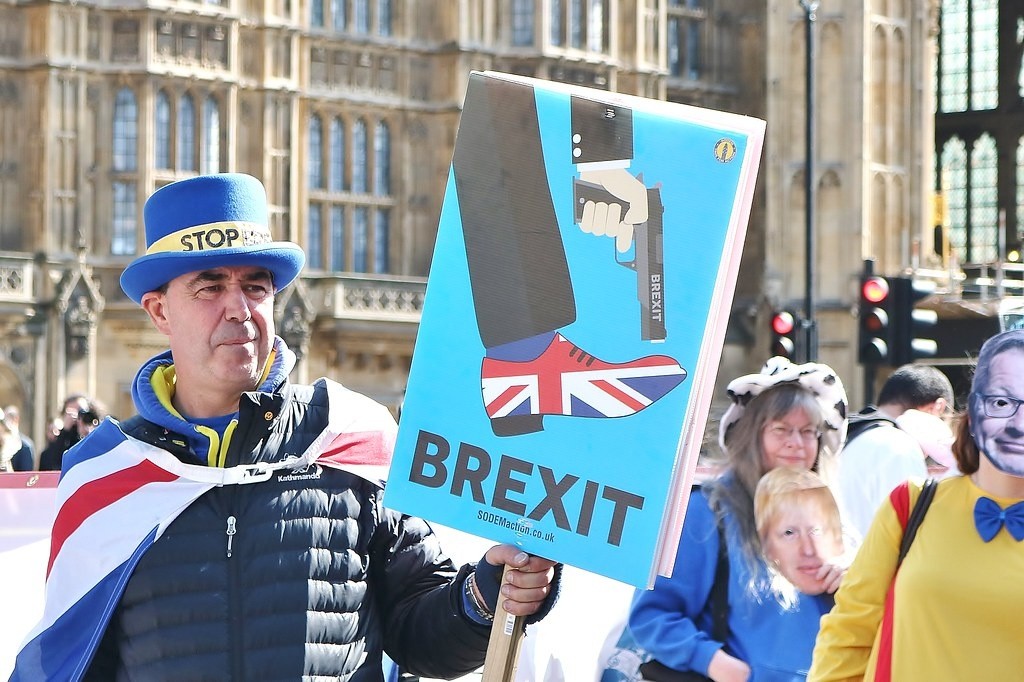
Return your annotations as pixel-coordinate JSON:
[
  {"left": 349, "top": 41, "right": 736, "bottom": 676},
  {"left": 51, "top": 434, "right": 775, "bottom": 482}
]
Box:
[{"left": 466, "top": 579, "right": 494, "bottom": 621}]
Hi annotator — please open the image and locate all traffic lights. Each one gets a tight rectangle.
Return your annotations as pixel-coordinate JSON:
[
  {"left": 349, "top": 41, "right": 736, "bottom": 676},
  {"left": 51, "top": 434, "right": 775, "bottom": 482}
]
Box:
[
  {"left": 893, "top": 277, "right": 938, "bottom": 366},
  {"left": 769, "top": 309, "right": 806, "bottom": 364},
  {"left": 859, "top": 275, "right": 894, "bottom": 365}
]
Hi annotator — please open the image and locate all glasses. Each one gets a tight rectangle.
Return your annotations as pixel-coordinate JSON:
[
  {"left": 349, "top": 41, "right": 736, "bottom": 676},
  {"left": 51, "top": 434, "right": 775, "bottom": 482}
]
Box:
[
  {"left": 975, "top": 392, "right": 1024, "bottom": 418},
  {"left": 769, "top": 421, "right": 824, "bottom": 442}
]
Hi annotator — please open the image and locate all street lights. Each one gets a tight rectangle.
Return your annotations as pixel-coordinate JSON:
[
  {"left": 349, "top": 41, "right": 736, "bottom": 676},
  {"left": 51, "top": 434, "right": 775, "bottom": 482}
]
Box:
[{"left": 801, "top": 1, "right": 818, "bottom": 319}]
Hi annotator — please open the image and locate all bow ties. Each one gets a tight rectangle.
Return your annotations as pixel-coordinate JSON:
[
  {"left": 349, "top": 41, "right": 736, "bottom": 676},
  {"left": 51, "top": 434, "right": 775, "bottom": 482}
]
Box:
[{"left": 974, "top": 497, "right": 1024, "bottom": 542}]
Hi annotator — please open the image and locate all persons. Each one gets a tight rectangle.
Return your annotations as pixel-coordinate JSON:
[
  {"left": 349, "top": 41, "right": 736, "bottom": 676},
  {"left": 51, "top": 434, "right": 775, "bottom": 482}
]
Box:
[
  {"left": 41, "top": 173, "right": 563, "bottom": 682},
  {"left": 630, "top": 356, "right": 957, "bottom": 682},
  {"left": 806, "top": 331, "right": 1024, "bottom": 682},
  {"left": 38, "top": 394, "right": 106, "bottom": 471},
  {"left": 0, "top": 406, "right": 36, "bottom": 471}
]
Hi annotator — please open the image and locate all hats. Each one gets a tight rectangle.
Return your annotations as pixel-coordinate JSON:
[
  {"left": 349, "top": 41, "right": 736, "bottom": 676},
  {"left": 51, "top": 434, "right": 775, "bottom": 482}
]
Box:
[
  {"left": 119, "top": 174, "right": 307, "bottom": 310},
  {"left": 718, "top": 357, "right": 849, "bottom": 477}
]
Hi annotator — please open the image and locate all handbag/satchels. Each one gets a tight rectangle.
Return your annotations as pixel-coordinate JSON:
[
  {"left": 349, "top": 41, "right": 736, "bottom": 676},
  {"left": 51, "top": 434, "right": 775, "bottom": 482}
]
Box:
[{"left": 638, "top": 484, "right": 731, "bottom": 680}]
